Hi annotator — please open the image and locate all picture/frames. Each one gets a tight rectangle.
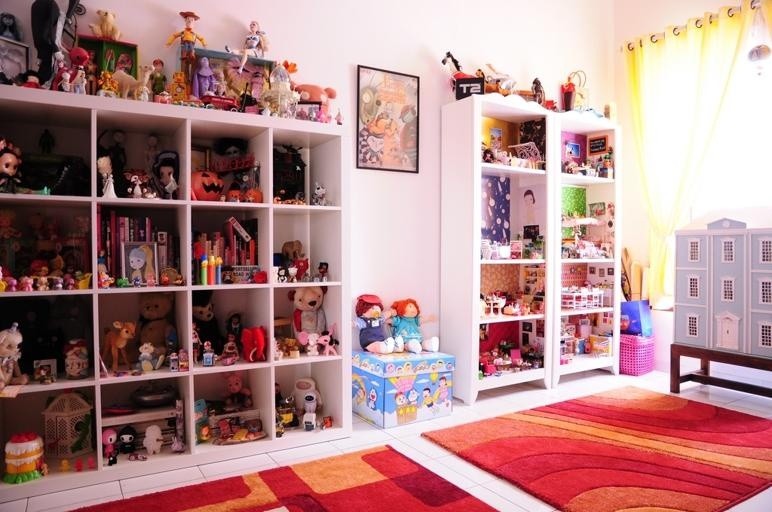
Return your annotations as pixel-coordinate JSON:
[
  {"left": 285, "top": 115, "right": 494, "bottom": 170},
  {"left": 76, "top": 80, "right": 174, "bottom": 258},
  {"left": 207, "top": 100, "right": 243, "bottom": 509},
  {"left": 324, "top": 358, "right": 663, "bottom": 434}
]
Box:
[
  {"left": 181, "top": 48, "right": 277, "bottom": 79},
  {"left": 586, "top": 134, "right": 609, "bottom": 156},
  {"left": 356, "top": 64, "right": 421, "bottom": 174},
  {"left": 0, "top": 35, "right": 33, "bottom": 81},
  {"left": 119, "top": 238, "right": 161, "bottom": 286}
]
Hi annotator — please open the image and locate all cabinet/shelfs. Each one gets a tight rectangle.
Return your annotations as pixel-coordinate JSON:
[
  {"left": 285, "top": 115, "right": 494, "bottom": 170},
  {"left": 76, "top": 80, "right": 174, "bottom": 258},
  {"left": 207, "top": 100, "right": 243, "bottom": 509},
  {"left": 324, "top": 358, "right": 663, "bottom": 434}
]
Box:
[
  {"left": 669, "top": 341, "right": 771, "bottom": 395},
  {"left": 439, "top": 95, "right": 624, "bottom": 407},
  {"left": 1, "top": 82, "right": 353, "bottom": 501}
]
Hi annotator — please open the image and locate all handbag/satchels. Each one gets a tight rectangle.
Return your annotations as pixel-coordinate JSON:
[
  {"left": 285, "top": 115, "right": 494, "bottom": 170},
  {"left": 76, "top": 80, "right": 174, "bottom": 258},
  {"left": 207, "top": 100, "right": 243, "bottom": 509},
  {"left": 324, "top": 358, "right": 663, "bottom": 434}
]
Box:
[{"left": 620, "top": 301, "right": 652, "bottom": 336}]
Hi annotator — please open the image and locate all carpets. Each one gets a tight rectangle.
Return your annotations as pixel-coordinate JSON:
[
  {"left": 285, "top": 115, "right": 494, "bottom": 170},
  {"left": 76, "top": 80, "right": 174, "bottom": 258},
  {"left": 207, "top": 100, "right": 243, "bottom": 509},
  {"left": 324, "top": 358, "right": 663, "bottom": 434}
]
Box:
[
  {"left": 66, "top": 439, "right": 500, "bottom": 512},
  {"left": 420, "top": 384, "right": 772, "bottom": 512}
]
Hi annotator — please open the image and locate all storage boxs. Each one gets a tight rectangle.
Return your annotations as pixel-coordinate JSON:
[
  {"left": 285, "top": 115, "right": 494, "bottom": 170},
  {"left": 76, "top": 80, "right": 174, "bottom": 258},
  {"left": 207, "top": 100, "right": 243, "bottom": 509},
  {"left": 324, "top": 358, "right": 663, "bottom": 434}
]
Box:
[{"left": 351, "top": 348, "right": 455, "bottom": 427}]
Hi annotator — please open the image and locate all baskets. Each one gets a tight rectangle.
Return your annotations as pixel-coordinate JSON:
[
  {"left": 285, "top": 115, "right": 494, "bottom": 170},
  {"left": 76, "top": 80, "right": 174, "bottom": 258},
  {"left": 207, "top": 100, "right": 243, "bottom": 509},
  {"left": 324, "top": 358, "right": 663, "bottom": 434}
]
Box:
[{"left": 620, "top": 334, "right": 656, "bottom": 376}]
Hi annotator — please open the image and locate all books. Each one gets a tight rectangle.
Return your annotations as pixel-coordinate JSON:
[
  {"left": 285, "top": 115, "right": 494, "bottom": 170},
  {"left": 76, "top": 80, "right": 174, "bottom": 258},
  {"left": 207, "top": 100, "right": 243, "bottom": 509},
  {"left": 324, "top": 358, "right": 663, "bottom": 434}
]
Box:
[{"left": 95, "top": 207, "right": 257, "bottom": 288}]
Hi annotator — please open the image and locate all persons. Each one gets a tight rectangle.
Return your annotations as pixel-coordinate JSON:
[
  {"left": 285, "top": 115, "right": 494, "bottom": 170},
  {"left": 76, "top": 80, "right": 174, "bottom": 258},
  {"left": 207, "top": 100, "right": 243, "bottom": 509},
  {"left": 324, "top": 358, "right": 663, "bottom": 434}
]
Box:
[
  {"left": 422, "top": 386, "right": 434, "bottom": 411},
  {"left": 386, "top": 298, "right": 440, "bottom": 354},
  {"left": 435, "top": 377, "right": 451, "bottom": 413},
  {"left": 148, "top": 11, "right": 268, "bottom": 113},
  {"left": 352, "top": 294, "right": 405, "bottom": 353}
]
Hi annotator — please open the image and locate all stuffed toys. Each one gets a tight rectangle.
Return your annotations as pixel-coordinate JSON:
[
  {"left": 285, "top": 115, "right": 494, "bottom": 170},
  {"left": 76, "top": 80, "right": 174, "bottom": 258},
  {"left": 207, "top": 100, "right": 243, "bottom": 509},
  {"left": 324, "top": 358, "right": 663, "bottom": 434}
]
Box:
[
  {"left": 2, "top": 107, "right": 181, "bottom": 201},
  {"left": 0, "top": 0, "right": 347, "bottom": 483},
  {"left": 1, "top": 286, "right": 340, "bottom": 483}
]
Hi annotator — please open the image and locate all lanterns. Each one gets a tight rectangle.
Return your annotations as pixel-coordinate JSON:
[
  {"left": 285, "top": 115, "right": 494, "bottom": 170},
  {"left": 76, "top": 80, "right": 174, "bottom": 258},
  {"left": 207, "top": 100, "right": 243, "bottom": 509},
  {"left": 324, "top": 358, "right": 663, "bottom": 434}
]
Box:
[{"left": 192, "top": 166, "right": 262, "bottom": 202}]
